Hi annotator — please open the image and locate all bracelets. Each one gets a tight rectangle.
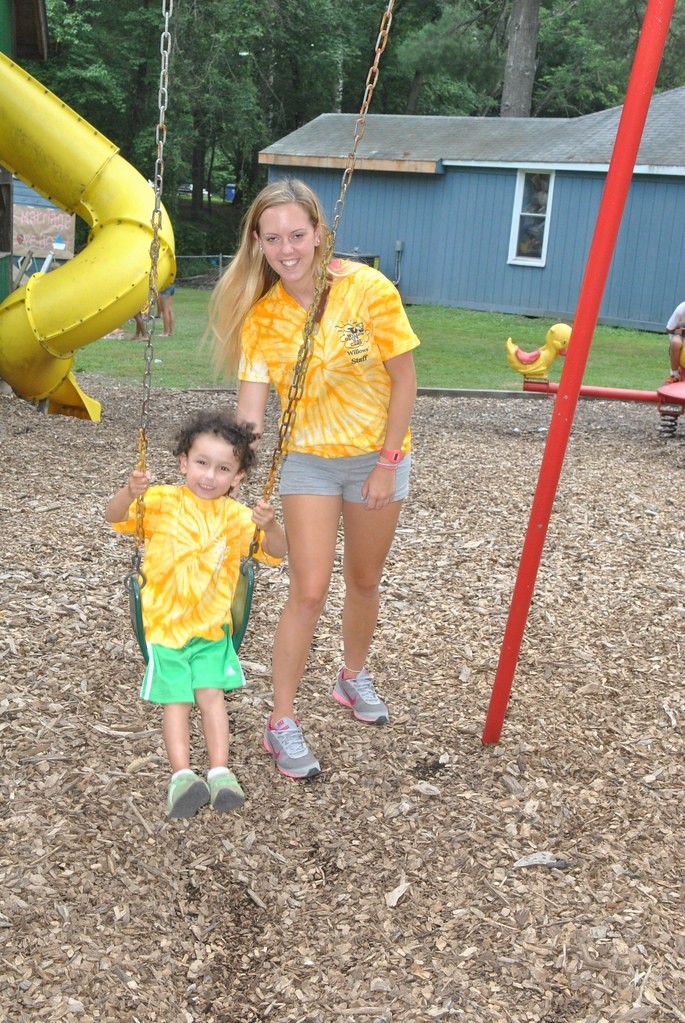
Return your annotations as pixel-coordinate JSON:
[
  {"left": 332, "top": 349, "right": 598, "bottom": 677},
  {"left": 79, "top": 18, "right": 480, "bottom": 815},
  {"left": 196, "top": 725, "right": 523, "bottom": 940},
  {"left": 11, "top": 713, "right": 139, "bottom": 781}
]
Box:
[
  {"left": 376, "top": 461, "right": 397, "bottom": 466},
  {"left": 375, "top": 463, "right": 398, "bottom": 470}
]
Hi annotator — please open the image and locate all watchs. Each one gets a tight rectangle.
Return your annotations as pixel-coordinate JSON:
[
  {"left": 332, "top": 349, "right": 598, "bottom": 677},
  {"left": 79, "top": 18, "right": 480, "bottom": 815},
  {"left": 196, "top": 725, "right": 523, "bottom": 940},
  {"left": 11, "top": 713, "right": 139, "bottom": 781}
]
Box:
[{"left": 379, "top": 447, "right": 403, "bottom": 463}]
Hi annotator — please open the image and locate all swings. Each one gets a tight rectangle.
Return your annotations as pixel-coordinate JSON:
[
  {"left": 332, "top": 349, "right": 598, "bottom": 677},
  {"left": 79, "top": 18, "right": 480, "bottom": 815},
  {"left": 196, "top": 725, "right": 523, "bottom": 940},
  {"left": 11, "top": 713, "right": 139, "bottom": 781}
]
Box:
[{"left": 124, "top": 0, "right": 392, "bottom": 665}]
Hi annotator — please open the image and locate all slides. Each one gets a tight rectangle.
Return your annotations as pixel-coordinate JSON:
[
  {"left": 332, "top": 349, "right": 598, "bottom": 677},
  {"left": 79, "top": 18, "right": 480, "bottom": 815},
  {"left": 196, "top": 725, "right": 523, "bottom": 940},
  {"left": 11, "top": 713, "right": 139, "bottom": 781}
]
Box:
[{"left": 1, "top": 50, "right": 177, "bottom": 425}]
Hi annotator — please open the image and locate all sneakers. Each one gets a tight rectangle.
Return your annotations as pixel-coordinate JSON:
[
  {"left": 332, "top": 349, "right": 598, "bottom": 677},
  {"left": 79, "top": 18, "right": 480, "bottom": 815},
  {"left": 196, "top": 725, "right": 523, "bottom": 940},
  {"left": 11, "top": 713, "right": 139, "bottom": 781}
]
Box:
[
  {"left": 262, "top": 716, "right": 321, "bottom": 778},
  {"left": 209, "top": 771, "right": 246, "bottom": 811},
  {"left": 331, "top": 667, "right": 391, "bottom": 724},
  {"left": 662, "top": 375, "right": 681, "bottom": 387},
  {"left": 165, "top": 775, "right": 211, "bottom": 820}
]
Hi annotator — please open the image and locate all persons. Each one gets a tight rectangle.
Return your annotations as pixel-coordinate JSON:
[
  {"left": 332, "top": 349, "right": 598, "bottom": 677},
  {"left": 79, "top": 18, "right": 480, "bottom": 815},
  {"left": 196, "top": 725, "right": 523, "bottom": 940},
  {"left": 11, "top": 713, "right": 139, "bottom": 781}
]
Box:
[
  {"left": 131, "top": 312, "right": 150, "bottom": 341},
  {"left": 159, "top": 285, "right": 174, "bottom": 337},
  {"left": 105, "top": 411, "right": 288, "bottom": 817},
  {"left": 153, "top": 297, "right": 162, "bottom": 318},
  {"left": 200, "top": 180, "right": 422, "bottom": 779},
  {"left": 665, "top": 301, "right": 685, "bottom": 384}
]
